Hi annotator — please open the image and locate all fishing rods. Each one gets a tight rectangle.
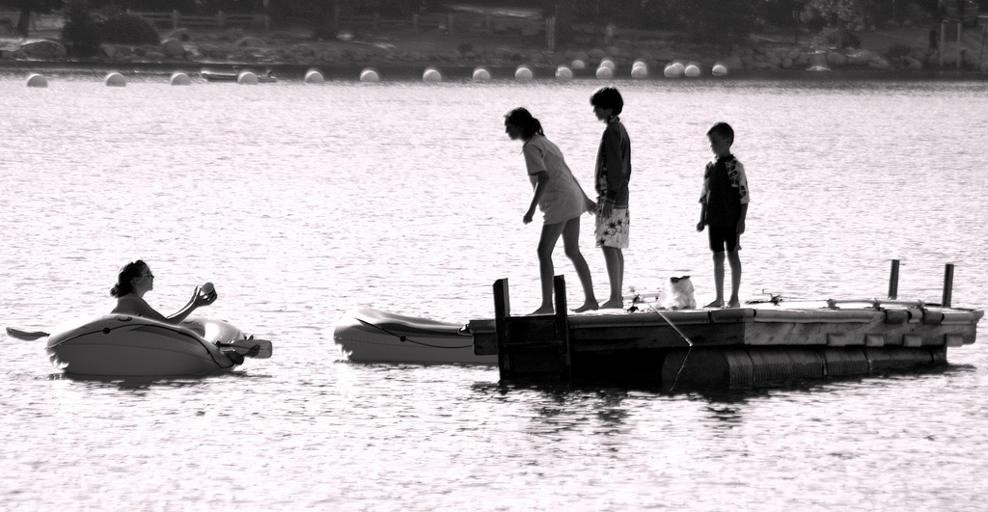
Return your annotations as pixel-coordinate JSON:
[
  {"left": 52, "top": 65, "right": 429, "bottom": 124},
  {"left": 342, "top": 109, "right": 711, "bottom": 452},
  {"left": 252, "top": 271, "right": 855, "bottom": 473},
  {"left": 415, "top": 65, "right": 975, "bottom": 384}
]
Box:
[
  {"left": 628, "top": 286, "right": 697, "bottom": 351},
  {"left": 746, "top": 288, "right": 974, "bottom": 312}
]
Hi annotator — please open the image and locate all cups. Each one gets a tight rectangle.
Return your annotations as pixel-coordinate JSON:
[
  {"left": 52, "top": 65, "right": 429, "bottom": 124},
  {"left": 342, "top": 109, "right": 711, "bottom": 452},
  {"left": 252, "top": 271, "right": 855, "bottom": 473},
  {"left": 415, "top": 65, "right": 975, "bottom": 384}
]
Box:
[{"left": 202, "top": 282, "right": 218, "bottom": 299}]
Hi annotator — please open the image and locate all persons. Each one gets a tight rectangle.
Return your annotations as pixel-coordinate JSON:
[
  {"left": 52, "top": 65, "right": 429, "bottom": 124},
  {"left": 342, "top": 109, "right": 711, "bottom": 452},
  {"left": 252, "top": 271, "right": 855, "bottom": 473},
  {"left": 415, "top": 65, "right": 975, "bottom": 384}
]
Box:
[
  {"left": 110, "top": 259, "right": 217, "bottom": 337},
  {"left": 589, "top": 86, "right": 631, "bottom": 308},
  {"left": 504, "top": 107, "right": 599, "bottom": 315},
  {"left": 696, "top": 122, "right": 750, "bottom": 309}
]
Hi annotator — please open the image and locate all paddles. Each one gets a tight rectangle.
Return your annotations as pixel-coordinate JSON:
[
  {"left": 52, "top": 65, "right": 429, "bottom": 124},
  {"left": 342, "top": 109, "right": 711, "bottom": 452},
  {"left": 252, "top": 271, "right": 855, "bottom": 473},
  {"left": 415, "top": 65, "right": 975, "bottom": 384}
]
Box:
[{"left": 6, "top": 327, "right": 274, "bottom": 357}]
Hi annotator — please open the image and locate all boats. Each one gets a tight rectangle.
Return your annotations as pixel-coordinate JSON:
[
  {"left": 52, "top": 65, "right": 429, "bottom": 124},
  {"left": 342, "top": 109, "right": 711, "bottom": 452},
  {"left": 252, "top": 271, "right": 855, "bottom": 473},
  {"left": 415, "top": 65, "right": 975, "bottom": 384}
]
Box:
[
  {"left": 47, "top": 312, "right": 248, "bottom": 378},
  {"left": 332, "top": 302, "right": 784, "bottom": 391},
  {"left": 461, "top": 259, "right": 985, "bottom": 408}
]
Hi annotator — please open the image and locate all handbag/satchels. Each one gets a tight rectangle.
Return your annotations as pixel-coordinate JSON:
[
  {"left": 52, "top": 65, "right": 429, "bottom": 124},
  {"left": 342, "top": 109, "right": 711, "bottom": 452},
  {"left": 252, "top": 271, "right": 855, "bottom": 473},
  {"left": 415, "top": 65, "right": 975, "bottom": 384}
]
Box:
[{"left": 655, "top": 274, "right": 697, "bottom": 310}]
